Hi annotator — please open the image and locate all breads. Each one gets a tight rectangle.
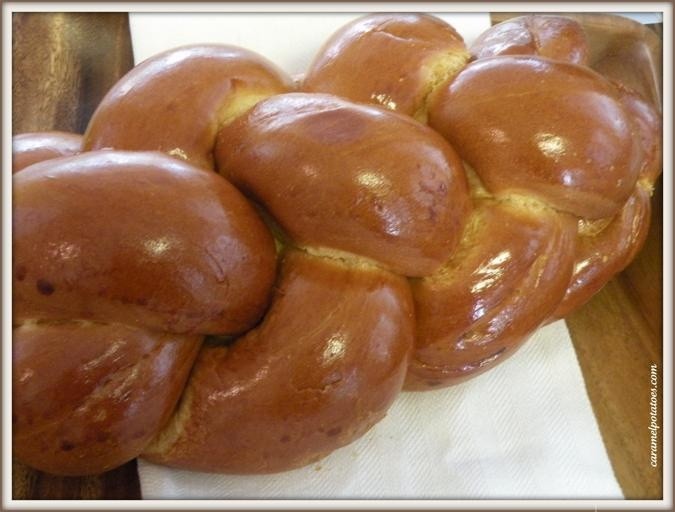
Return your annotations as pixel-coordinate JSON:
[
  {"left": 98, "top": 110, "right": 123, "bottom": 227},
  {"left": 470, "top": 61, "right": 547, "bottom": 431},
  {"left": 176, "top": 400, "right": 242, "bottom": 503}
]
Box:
[{"left": 12, "top": 12, "right": 662, "bottom": 474}]
